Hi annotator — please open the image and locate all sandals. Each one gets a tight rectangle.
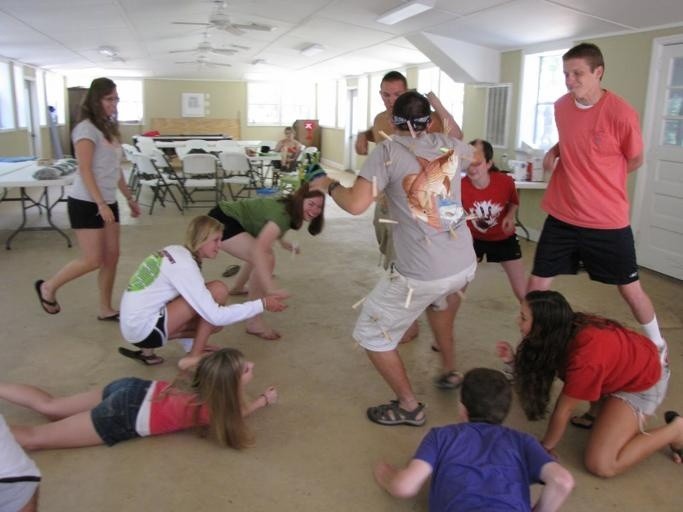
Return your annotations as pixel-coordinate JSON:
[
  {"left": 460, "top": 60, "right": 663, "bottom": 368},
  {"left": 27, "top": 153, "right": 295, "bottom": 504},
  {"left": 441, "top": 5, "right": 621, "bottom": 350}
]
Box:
[
  {"left": 367, "top": 398, "right": 428, "bottom": 427},
  {"left": 431, "top": 371, "right": 465, "bottom": 390},
  {"left": 664, "top": 411, "right": 683, "bottom": 465}
]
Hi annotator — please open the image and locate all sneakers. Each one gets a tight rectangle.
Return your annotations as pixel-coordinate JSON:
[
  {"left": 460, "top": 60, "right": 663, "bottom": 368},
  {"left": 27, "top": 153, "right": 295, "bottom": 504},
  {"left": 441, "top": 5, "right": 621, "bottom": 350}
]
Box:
[{"left": 659, "top": 336, "right": 671, "bottom": 381}]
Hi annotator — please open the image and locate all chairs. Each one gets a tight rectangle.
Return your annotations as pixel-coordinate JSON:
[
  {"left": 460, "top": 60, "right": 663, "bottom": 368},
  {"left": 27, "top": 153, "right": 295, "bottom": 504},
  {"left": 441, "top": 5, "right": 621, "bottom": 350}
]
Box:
[
  {"left": 131, "top": 151, "right": 184, "bottom": 215},
  {"left": 215, "top": 140, "right": 242, "bottom": 148},
  {"left": 182, "top": 155, "right": 219, "bottom": 208},
  {"left": 132, "top": 136, "right": 175, "bottom": 166},
  {"left": 188, "top": 139, "right": 213, "bottom": 146},
  {"left": 220, "top": 153, "right": 258, "bottom": 204},
  {"left": 295, "top": 145, "right": 306, "bottom": 161},
  {"left": 175, "top": 141, "right": 195, "bottom": 157},
  {"left": 273, "top": 146, "right": 317, "bottom": 187},
  {"left": 120, "top": 142, "right": 167, "bottom": 188}
]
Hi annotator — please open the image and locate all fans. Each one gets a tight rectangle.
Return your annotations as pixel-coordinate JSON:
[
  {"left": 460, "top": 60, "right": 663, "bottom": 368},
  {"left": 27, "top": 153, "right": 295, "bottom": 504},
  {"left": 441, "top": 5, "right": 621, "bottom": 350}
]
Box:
[
  {"left": 169, "top": 33, "right": 240, "bottom": 55},
  {"left": 175, "top": 56, "right": 235, "bottom": 68},
  {"left": 173, "top": 2, "right": 276, "bottom": 34}
]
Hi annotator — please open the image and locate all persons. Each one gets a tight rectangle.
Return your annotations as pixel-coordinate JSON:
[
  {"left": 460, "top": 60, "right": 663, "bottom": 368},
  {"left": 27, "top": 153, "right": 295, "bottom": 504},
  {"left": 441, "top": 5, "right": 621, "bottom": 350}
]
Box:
[
  {"left": 495, "top": 291, "right": 683, "bottom": 477},
  {"left": 48, "top": 105, "right": 57, "bottom": 124},
  {"left": 206, "top": 182, "right": 326, "bottom": 340},
  {"left": 121, "top": 216, "right": 291, "bottom": 369},
  {"left": 0, "top": 416, "right": 41, "bottom": 512},
  {"left": 370, "top": 368, "right": 575, "bottom": 512},
  {"left": 356, "top": 70, "right": 463, "bottom": 345},
  {"left": 0, "top": 348, "right": 277, "bottom": 451},
  {"left": 527, "top": 43, "right": 668, "bottom": 370},
  {"left": 309, "top": 92, "right": 478, "bottom": 425},
  {"left": 37, "top": 77, "right": 142, "bottom": 322},
  {"left": 432, "top": 139, "right": 530, "bottom": 351},
  {"left": 276, "top": 127, "right": 303, "bottom": 154}
]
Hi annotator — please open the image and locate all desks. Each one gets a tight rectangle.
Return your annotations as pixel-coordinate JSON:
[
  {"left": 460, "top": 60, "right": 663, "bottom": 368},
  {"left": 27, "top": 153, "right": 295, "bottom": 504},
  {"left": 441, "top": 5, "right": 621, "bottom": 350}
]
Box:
[
  {"left": 459, "top": 171, "right": 548, "bottom": 241},
  {"left": 2, "top": 158, "right": 78, "bottom": 250},
  {"left": 0, "top": 162, "right": 42, "bottom": 217}
]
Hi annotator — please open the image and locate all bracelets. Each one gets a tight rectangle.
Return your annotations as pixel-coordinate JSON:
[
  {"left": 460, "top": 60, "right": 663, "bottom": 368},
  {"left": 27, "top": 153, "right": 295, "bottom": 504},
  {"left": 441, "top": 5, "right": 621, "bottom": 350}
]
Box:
[
  {"left": 263, "top": 297, "right": 267, "bottom": 311},
  {"left": 127, "top": 196, "right": 133, "bottom": 201},
  {"left": 259, "top": 393, "right": 268, "bottom": 407},
  {"left": 96, "top": 200, "right": 108, "bottom": 217}
]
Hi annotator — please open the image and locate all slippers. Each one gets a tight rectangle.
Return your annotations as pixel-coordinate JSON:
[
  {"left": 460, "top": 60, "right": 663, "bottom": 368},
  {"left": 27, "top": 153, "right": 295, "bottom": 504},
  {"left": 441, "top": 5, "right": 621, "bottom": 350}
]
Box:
[
  {"left": 118, "top": 345, "right": 164, "bottom": 365},
  {"left": 97, "top": 310, "right": 121, "bottom": 321},
  {"left": 34, "top": 279, "right": 62, "bottom": 315},
  {"left": 570, "top": 412, "right": 596, "bottom": 429},
  {"left": 222, "top": 264, "right": 241, "bottom": 278}
]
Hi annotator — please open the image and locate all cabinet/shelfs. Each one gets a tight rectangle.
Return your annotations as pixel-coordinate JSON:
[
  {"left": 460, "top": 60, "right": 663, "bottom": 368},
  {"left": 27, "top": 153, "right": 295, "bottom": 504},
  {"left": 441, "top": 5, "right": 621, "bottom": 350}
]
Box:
[{"left": 67, "top": 87, "right": 91, "bottom": 156}]
